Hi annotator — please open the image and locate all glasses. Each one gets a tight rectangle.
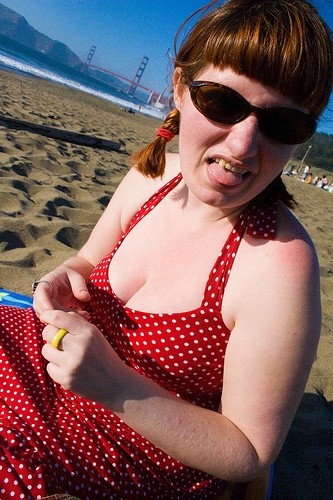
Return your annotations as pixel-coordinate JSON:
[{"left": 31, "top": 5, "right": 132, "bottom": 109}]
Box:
[{"left": 184, "top": 77, "right": 317, "bottom": 146}]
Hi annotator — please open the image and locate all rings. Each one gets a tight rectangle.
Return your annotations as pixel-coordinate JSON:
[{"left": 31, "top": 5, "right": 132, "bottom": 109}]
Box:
[{"left": 49, "top": 326, "right": 65, "bottom": 350}]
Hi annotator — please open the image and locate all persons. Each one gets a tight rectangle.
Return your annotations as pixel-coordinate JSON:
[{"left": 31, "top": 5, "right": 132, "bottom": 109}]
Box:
[
  {"left": 301, "top": 164, "right": 327, "bottom": 189},
  {"left": 1, "top": 0, "right": 333, "bottom": 500}
]
